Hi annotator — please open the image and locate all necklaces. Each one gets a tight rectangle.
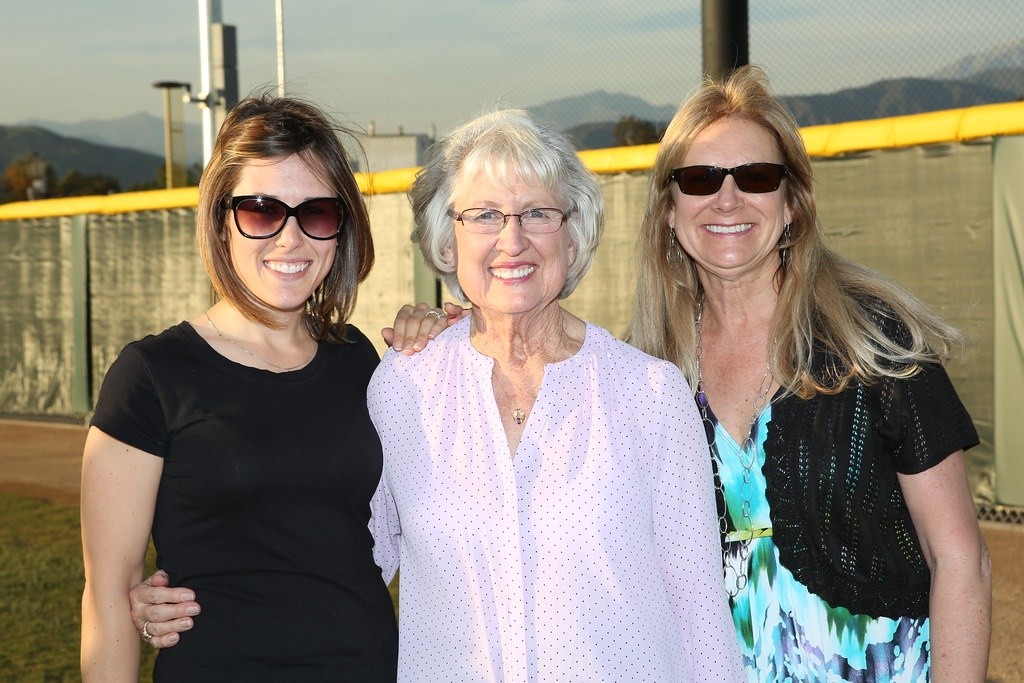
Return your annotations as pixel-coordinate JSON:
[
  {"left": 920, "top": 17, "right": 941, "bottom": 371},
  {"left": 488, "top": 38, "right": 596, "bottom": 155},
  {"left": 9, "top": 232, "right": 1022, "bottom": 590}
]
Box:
[
  {"left": 689, "top": 272, "right": 777, "bottom": 597},
  {"left": 202, "top": 304, "right": 317, "bottom": 371},
  {"left": 492, "top": 312, "right": 565, "bottom": 423}
]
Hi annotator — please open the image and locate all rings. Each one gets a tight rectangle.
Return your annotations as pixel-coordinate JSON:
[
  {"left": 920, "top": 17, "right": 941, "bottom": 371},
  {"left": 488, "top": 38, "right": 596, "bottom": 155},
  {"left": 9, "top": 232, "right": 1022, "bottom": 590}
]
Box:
[
  {"left": 142, "top": 620, "right": 157, "bottom": 641},
  {"left": 425, "top": 310, "right": 441, "bottom": 319},
  {"left": 442, "top": 313, "right": 449, "bottom": 318}
]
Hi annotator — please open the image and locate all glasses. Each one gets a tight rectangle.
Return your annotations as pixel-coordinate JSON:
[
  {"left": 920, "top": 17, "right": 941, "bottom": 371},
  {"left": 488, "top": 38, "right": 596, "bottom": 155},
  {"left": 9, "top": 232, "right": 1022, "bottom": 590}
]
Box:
[
  {"left": 220, "top": 195, "right": 349, "bottom": 240},
  {"left": 669, "top": 163, "right": 789, "bottom": 196},
  {"left": 448, "top": 204, "right": 577, "bottom": 234}
]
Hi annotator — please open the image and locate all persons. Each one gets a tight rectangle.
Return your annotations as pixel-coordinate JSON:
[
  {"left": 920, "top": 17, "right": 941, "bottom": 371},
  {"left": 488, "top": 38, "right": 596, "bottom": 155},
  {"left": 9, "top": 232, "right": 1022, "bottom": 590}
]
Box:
[
  {"left": 127, "top": 109, "right": 744, "bottom": 682},
  {"left": 639, "top": 65, "right": 993, "bottom": 682},
  {"left": 82, "top": 97, "right": 461, "bottom": 682}
]
